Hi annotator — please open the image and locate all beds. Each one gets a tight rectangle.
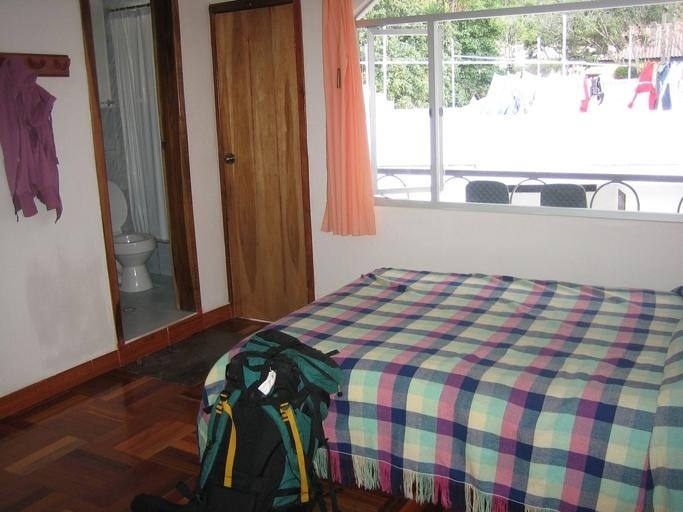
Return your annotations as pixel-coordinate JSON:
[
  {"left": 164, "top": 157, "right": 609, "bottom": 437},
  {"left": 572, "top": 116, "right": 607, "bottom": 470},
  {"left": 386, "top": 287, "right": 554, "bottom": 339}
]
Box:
[{"left": 197, "top": 266, "right": 683, "bottom": 511}]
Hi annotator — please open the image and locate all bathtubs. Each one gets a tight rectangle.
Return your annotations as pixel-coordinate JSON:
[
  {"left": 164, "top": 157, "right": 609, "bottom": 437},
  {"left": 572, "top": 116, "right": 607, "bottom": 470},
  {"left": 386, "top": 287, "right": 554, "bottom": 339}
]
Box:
[{"left": 146, "top": 239, "right": 173, "bottom": 276}]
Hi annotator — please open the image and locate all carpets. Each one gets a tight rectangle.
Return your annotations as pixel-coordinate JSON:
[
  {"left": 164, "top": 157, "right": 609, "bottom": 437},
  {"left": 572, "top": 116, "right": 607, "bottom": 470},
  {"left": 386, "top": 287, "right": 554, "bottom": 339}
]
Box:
[{"left": 123, "top": 328, "right": 245, "bottom": 390}]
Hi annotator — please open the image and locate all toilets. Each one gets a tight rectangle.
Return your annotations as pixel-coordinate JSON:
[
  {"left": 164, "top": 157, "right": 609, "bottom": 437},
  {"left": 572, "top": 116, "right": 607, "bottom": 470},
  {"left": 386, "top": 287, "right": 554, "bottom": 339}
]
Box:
[{"left": 108, "top": 180, "right": 157, "bottom": 293}]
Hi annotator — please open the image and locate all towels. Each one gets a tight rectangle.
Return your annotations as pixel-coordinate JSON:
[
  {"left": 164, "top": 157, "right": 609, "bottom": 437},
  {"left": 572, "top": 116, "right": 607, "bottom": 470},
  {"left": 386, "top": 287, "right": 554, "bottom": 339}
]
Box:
[{"left": 0, "top": 56, "right": 62, "bottom": 223}]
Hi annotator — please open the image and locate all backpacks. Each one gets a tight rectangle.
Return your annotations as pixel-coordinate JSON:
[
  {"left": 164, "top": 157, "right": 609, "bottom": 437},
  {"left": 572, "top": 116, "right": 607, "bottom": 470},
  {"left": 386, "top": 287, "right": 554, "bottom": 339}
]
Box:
[{"left": 186, "top": 330, "right": 344, "bottom": 512}]
